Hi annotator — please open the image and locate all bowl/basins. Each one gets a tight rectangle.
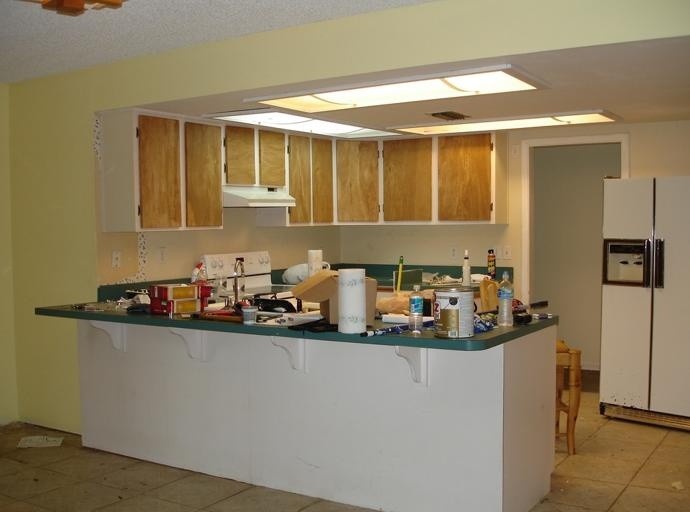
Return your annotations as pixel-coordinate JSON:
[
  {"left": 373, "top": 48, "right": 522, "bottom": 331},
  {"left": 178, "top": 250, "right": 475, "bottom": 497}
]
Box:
[{"left": 515, "top": 312, "right": 532, "bottom": 325}]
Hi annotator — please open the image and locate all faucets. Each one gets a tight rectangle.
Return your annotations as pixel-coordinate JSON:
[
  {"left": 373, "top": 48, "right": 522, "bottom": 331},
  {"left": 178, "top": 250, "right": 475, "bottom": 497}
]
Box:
[{"left": 234, "top": 260, "right": 245, "bottom": 304}]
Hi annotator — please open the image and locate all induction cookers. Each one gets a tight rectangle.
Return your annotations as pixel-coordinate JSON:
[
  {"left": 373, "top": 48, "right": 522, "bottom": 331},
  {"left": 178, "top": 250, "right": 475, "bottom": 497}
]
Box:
[{"left": 226, "top": 284, "right": 301, "bottom": 296}]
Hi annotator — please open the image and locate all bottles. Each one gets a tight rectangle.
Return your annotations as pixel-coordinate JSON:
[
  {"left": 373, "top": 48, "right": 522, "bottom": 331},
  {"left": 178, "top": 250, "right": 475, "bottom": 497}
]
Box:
[
  {"left": 461, "top": 251, "right": 471, "bottom": 288},
  {"left": 409, "top": 284, "right": 424, "bottom": 334},
  {"left": 495, "top": 270, "right": 514, "bottom": 329},
  {"left": 487, "top": 249, "right": 496, "bottom": 279},
  {"left": 307, "top": 250, "right": 323, "bottom": 280}
]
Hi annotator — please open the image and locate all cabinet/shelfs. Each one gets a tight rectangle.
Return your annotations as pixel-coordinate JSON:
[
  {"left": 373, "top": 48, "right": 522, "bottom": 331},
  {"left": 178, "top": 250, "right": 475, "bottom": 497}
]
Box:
[
  {"left": 334, "top": 139, "right": 381, "bottom": 227},
  {"left": 223, "top": 124, "right": 285, "bottom": 189},
  {"left": 97, "top": 109, "right": 224, "bottom": 234},
  {"left": 381, "top": 134, "right": 508, "bottom": 226},
  {"left": 254, "top": 134, "right": 335, "bottom": 228}
]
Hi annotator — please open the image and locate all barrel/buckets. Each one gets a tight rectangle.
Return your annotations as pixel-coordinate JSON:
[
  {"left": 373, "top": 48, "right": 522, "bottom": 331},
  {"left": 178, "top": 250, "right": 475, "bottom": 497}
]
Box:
[{"left": 434, "top": 287, "right": 474, "bottom": 338}]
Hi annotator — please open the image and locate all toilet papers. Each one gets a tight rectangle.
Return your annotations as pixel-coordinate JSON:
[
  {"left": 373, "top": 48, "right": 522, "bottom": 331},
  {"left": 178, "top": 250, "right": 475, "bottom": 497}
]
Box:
[
  {"left": 338, "top": 268, "right": 366, "bottom": 334},
  {"left": 308, "top": 249, "right": 322, "bottom": 279}
]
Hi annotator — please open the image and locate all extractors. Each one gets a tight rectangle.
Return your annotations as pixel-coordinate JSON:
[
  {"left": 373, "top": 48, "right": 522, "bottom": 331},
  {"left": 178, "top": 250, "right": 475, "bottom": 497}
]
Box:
[{"left": 223, "top": 186, "right": 296, "bottom": 208}]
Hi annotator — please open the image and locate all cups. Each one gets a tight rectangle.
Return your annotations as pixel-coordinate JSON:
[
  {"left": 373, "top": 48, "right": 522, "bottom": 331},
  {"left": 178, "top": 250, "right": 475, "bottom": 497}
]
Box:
[{"left": 240, "top": 306, "right": 258, "bottom": 325}]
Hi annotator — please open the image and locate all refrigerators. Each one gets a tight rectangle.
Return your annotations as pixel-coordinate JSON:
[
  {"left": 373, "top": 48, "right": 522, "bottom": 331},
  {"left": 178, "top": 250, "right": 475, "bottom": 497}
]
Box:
[{"left": 601, "top": 177, "right": 690, "bottom": 420}]
